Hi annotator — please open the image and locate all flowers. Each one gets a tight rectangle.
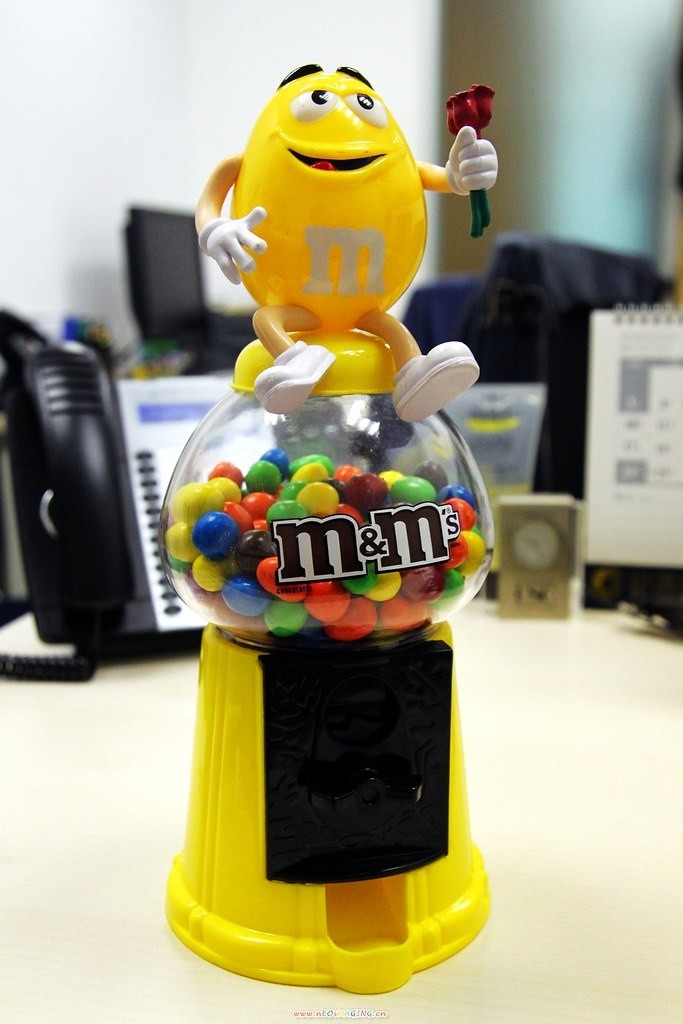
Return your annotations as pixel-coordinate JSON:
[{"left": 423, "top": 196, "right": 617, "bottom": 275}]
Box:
[{"left": 444, "top": 83, "right": 497, "bottom": 238}]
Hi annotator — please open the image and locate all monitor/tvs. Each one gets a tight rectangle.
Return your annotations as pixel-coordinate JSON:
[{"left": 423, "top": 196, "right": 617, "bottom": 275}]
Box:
[{"left": 126, "top": 207, "right": 205, "bottom": 344}]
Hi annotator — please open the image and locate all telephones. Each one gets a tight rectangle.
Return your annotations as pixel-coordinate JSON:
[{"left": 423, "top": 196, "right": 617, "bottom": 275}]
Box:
[{"left": 0, "top": 342, "right": 284, "bottom": 682}]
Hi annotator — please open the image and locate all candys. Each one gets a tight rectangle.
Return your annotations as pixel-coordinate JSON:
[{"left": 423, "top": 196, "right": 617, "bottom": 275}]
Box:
[{"left": 166, "top": 448, "right": 489, "bottom": 640}]
